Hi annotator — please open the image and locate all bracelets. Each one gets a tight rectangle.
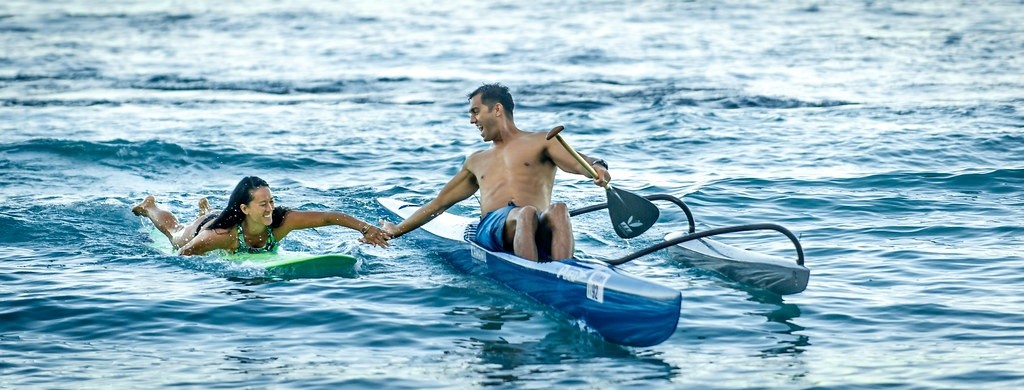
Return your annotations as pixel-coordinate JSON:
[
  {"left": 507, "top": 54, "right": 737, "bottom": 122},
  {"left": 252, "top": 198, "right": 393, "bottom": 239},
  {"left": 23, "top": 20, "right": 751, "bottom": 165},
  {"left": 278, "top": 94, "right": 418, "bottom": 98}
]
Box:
[{"left": 361, "top": 223, "right": 372, "bottom": 235}]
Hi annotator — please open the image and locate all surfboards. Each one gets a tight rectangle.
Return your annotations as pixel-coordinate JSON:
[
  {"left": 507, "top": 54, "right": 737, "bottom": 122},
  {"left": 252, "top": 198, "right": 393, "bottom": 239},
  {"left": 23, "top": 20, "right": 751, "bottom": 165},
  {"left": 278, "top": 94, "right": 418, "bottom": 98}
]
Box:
[{"left": 142, "top": 227, "right": 358, "bottom": 284}]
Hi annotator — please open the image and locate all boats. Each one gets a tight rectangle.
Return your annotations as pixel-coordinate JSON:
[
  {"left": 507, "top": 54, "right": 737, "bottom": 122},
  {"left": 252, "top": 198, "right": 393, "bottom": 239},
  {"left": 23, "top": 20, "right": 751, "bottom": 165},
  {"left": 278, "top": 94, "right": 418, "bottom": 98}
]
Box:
[{"left": 375, "top": 195, "right": 810, "bottom": 347}]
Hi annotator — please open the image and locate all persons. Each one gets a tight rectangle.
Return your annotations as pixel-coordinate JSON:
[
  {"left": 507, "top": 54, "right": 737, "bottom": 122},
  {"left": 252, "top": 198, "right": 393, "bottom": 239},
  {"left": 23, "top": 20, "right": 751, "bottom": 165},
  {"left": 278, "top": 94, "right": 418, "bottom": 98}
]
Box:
[
  {"left": 132, "top": 176, "right": 394, "bottom": 256},
  {"left": 357, "top": 83, "right": 611, "bottom": 263}
]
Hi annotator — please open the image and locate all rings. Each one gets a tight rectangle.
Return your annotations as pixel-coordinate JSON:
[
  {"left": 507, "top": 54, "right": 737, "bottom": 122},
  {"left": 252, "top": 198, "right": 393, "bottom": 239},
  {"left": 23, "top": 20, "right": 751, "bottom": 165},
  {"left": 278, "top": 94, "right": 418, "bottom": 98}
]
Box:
[
  {"left": 604, "top": 179, "right": 608, "bottom": 183},
  {"left": 380, "top": 233, "right": 382, "bottom": 236}
]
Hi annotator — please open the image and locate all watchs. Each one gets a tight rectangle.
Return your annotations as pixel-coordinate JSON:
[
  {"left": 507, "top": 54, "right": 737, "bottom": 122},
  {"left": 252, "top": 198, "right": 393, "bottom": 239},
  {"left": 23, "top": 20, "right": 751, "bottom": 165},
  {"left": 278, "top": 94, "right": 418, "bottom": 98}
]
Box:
[{"left": 592, "top": 159, "right": 608, "bottom": 170}]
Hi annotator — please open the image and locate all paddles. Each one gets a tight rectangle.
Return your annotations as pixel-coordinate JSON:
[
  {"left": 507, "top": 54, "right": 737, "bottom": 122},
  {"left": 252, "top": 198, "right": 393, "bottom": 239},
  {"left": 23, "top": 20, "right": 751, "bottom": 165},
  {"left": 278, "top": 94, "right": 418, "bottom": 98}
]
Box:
[{"left": 545, "top": 123, "right": 661, "bottom": 241}]
